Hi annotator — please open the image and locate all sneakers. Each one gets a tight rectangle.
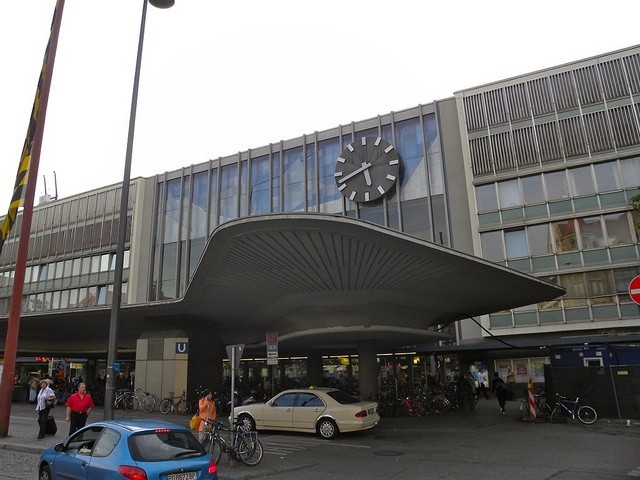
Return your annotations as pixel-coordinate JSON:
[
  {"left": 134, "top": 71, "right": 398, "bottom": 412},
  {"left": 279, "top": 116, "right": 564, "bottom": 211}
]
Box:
[{"left": 37, "top": 434, "right": 45, "bottom": 440}]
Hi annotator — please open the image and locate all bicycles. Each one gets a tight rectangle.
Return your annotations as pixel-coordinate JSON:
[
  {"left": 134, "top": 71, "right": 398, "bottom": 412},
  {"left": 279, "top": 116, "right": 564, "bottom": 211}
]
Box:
[
  {"left": 125, "top": 388, "right": 156, "bottom": 413},
  {"left": 201, "top": 417, "right": 263, "bottom": 465},
  {"left": 377, "top": 390, "right": 451, "bottom": 418},
  {"left": 47, "top": 380, "right": 105, "bottom": 405},
  {"left": 159, "top": 390, "right": 191, "bottom": 415},
  {"left": 192, "top": 386, "right": 229, "bottom": 418},
  {"left": 520, "top": 391, "right": 551, "bottom": 421},
  {"left": 550, "top": 393, "right": 597, "bottom": 425}
]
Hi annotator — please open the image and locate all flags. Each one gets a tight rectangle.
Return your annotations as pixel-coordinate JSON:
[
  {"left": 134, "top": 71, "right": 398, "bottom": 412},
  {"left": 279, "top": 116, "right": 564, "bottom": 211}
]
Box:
[{"left": 1, "top": 7, "right": 58, "bottom": 255}]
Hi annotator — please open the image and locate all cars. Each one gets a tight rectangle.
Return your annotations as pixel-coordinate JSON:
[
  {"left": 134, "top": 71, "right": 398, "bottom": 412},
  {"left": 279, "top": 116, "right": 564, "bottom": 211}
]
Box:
[
  {"left": 39, "top": 419, "right": 217, "bottom": 479},
  {"left": 228, "top": 386, "right": 380, "bottom": 439}
]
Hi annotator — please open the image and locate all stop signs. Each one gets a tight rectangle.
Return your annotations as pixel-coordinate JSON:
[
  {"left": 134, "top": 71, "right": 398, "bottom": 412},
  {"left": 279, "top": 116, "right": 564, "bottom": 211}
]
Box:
[{"left": 628, "top": 274, "right": 640, "bottom": 304}]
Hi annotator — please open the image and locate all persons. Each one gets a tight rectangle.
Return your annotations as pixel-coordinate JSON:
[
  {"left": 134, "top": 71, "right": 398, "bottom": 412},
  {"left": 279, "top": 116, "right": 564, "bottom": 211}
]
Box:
[
  {"left": 99, "top": 374, "right": 134, "bottom": 389},
  {"left": 476, "top": 368, "right": 489, "bottom": 399},
  {"left": 65, "top": 381, "right": 95, "bottom": 441},
  {"left": 489, "top": 372, "right": 507, "bottom": 413},
  {"left": 198, "top": 391, "right": 217, "bottom": 457},
  {"left": 34, "top": 380, "right": 58, "bottom": 439},
  {"left": 16, "top": 372, "right": 85, "bottom": 403},
  {"left": 506, "top": 365, "right": 517, "bottom": 399}
]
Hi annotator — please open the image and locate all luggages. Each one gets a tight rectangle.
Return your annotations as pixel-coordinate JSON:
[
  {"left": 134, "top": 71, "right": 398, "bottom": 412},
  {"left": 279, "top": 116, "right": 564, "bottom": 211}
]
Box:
[{"left": 38, "top": 416, "right": 57, "bottom": 435}]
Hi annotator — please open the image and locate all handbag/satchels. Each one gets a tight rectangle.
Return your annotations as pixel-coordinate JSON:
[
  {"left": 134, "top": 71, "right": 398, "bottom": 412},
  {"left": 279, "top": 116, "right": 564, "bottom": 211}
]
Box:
[
  {"left": 190, "top": 409, "right": 202, "bottom": 430},
  {"left": 45, "top": 399, "right": 56, "bottom": 407}
]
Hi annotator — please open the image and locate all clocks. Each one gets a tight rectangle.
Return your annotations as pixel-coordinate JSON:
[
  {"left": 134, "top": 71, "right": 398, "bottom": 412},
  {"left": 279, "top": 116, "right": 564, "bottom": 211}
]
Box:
[{"left": 334, "top": 137, "right": 401, "bottom": 203}]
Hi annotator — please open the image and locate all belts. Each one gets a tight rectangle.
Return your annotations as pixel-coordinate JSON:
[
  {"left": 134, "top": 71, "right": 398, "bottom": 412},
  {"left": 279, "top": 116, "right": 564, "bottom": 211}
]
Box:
[{"left": 74, "top": 411, "right": 87, "bottom": 414}]
